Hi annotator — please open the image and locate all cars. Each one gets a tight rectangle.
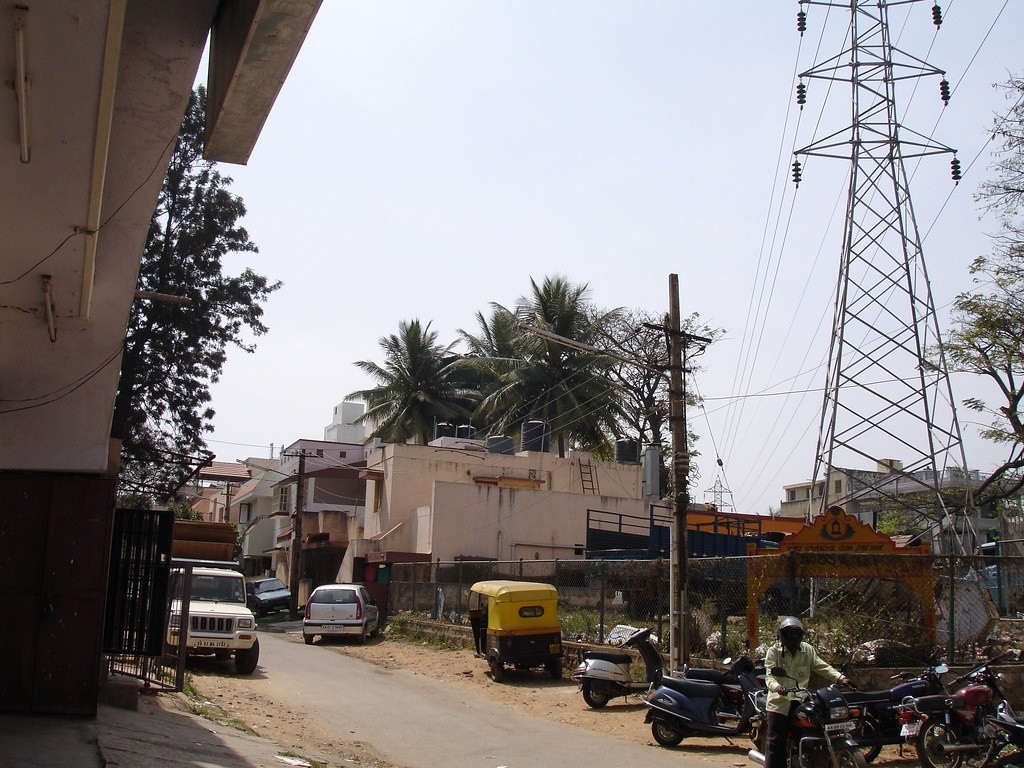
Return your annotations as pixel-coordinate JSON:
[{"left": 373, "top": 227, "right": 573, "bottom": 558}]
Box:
[
  {"left": 237, "top": 576, "right": 291, "bottom": 617},
  {"left": 302, "top": 584, "right": 379, "bottom": 645}
]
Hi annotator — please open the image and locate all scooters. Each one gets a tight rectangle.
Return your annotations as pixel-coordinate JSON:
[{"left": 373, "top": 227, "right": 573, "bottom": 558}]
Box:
[
  {"left": 639, "top": 657, "right": 767, "bottom": 748},
  {"left": 571, "top": 611, "right": 668, "bottom": 708}
]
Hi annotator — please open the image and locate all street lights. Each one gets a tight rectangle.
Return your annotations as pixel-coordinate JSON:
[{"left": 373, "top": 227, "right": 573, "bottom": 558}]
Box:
[
  {"left": 519, "top": 323, "right": 690, "bottom": 678},
  {"left": 529, "top": 419, "right": 553, "bottom": 452},
  {"left": 180, "top": 491, "right": 230, "bottom": 523},
  {"left": 236, "top": 459, "right": 304, "bottom": 622}
]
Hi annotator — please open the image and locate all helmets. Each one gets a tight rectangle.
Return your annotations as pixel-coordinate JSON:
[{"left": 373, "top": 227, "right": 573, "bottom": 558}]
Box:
[{"left": 779, "top": 616, "right": 804, "bottom": 648}]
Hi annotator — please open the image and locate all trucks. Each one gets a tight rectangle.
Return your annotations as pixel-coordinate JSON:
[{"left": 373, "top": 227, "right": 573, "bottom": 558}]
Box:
[{"left": 582, "top": 505, "right": 796, "bottom": 617}]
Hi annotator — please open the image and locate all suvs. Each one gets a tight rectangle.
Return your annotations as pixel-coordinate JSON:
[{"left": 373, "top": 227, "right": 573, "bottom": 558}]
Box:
[{"left": 167, "top": 567, "right": 259, "bottom": 675}]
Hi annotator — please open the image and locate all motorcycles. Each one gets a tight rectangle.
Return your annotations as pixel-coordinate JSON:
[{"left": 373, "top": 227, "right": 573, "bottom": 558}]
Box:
[
  {"left": 841, "top": 647, "right": 951, "bottom": 762},
  {"left": 748, "top": 663, "right": 867, "bottom": 768},
  {"left": 468, "top": 580, "right": 562, "bottom": 683},
  {"left": 886, "top": 647, "right": 1021, "bottom": 768},
  {"left": 673, "top": 634, "right": 767, "bottom": 723}
]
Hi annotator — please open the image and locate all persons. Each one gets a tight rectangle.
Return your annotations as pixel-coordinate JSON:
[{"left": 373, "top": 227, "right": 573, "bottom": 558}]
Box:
[{"left": 764, "top": 615, "right": 852, "bottom": 768}]
